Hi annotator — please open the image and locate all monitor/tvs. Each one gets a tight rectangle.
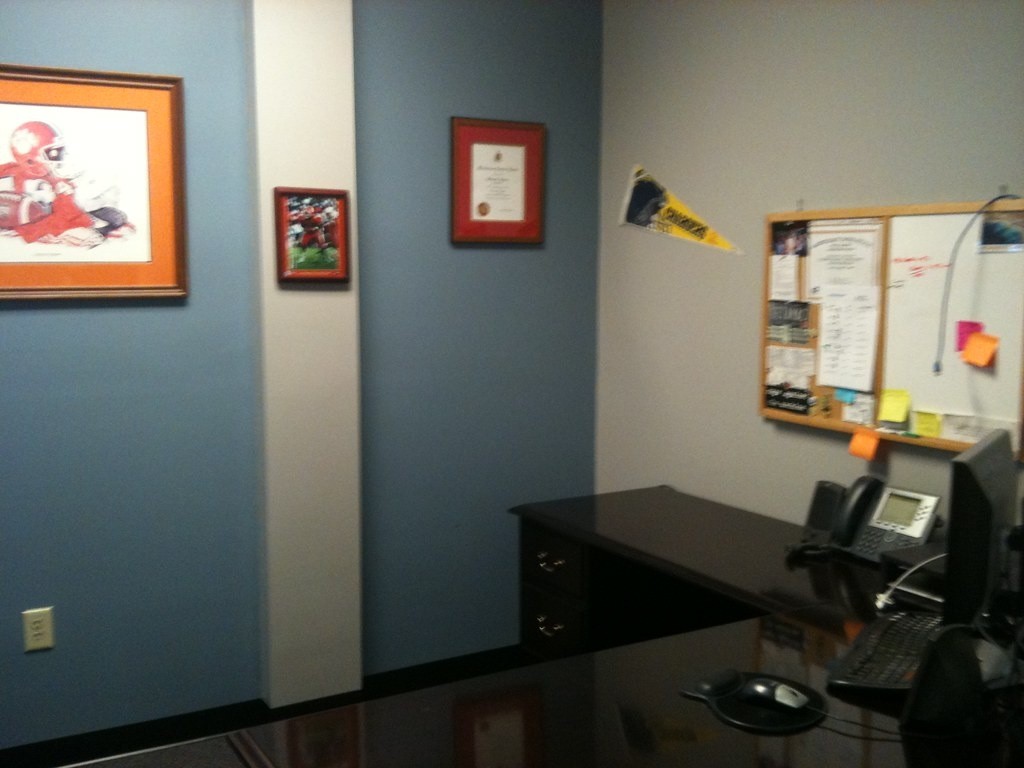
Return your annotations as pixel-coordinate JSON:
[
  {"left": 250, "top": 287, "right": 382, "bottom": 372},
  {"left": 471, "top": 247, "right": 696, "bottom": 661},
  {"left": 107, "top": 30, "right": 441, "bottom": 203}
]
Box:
[{"left": 940, "top": 429, "right": 1023, "bottom": 712}]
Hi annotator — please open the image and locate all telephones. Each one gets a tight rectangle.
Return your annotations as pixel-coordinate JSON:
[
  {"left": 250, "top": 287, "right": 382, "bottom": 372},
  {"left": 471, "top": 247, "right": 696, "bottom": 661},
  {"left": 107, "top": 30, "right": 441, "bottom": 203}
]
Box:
[{"left": 831, "top": 477, "right": 941, "bottom": 566}]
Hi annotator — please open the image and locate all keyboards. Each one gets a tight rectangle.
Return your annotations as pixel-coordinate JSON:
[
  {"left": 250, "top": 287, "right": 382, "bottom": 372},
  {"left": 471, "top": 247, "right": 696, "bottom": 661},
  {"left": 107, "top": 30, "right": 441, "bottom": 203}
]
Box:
[{"left": 826, "top": 610, "right": 964, "bottom": 694}]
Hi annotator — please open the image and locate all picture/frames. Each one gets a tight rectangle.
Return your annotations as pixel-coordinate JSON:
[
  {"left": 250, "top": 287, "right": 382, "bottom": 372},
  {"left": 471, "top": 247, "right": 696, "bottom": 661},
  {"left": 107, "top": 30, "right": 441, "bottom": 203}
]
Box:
[
  {"left": 0, "top": 63, "right": 190, "bottom": 306},
  {"left": 450, "top": 117, "right": 544, "bottom": 247},
  {"left": 285, "top": 704, "right": 360, "bottom": 768},
  {"left": 274, "top": 187, "right": 350, "bottom": 284},
  {"left": 449, "top": 686, "right": 540, "bottom": 768}
]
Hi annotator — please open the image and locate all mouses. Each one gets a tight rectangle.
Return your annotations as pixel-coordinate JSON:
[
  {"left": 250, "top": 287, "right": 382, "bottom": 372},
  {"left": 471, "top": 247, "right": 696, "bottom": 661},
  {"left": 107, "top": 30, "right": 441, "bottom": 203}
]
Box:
[{"left": 741, "top": 678, "right": 810, "bottom": 712}]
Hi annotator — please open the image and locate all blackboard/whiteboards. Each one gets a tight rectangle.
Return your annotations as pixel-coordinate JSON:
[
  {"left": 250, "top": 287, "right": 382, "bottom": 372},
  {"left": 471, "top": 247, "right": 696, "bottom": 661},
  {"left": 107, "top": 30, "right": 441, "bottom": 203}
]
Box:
[{"left": 759, "top": 201, "right": 1024, "bottom": 460}]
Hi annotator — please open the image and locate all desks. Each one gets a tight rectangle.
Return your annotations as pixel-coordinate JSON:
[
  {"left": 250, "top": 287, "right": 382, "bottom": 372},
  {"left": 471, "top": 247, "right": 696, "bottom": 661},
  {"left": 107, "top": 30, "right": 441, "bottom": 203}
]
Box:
[{"left": 61, "top": 482, "right": 1024, "bottom": 768}]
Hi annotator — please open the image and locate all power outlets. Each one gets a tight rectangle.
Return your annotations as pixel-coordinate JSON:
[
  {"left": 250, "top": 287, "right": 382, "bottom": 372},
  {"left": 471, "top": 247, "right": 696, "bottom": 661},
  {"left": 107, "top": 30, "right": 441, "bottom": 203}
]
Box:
[{"left": 22, "top": 606, "right": 55, "bottom": 654}]
